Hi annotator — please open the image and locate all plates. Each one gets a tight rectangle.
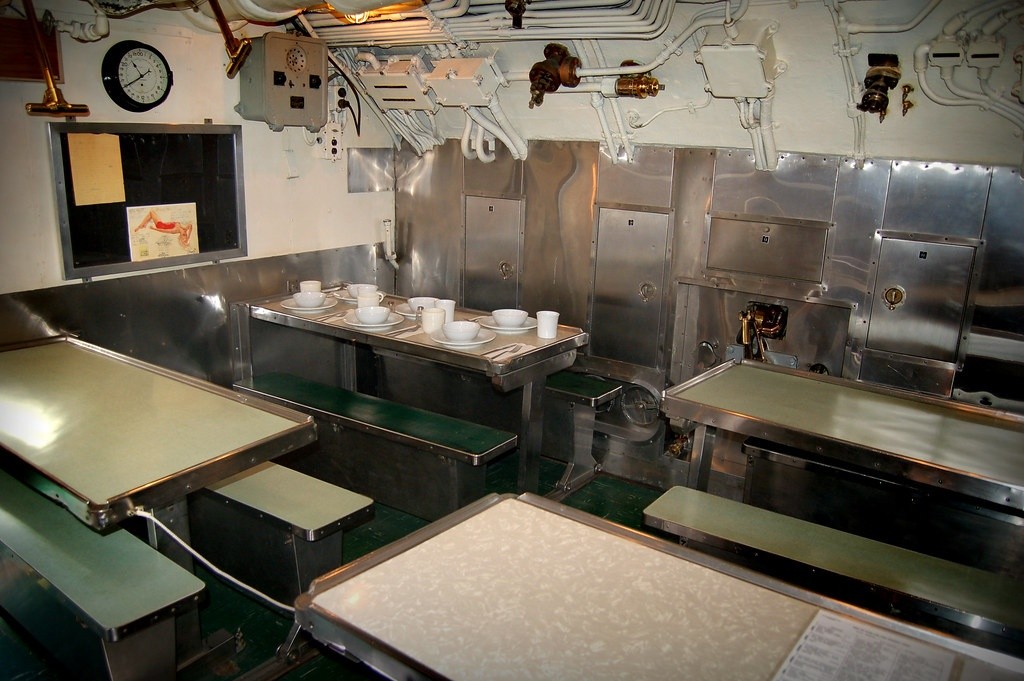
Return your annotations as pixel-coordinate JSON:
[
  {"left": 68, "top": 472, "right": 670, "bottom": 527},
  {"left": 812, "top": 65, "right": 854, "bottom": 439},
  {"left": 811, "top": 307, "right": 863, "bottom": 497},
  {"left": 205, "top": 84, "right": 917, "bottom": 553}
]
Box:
[
  {"left": 333, "top": 290, "right": 387, "bottom": 305},
  {"left": 343, "top": 312, "right": 404, "bottom": 330},
  {"left": 394, "top": 303, "right": 422, "bottom": 320},
  {"left": 478, "top": 316, "right": 537, "bottom": 333},
  {"left": 429, "top": 328, "right": 496, "bottom": 349},
  {"left": 280, "top": 297, "right": 338, "bottom": 313}
]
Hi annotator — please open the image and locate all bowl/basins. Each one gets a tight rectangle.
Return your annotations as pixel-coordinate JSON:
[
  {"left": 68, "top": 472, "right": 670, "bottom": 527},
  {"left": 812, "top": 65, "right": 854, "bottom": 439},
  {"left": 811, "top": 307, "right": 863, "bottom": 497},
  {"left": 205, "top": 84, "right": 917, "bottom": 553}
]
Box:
[
  {"left": 441, "top": 320, "right": 480, "bottom": 340},
  {"left": 347, "top": 284, "right": 378, "bottom": 298},
  {"left": 354, "top": 306, "right": 391, "bottom": 324},
  {"left": 491, "top": 308, "right": 529, "bottom": 328},
  {"left": 292, "top": 292, "right": 326, "bottom": 307},
  {"left": 407, "top": 297, "right": 439, "bottom": 313}
]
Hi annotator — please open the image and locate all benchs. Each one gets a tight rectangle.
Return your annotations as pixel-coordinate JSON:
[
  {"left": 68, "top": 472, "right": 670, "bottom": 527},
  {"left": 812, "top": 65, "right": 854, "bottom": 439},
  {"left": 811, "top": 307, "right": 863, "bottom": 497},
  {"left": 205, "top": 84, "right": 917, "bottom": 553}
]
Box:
[
  {"left": 234, "top": 371, "right": 519, "bottom": 516},
  {"left": 640, "top": 485, "right": 1024, "bottom": 647},
  {"left": 196, "top": 458, "right": 376, "bottom": 661},
  {"left": 369, "top": 345, "right": 625, "bottom": 490},
  {"left": 0, "top": 469, "right": 208, "bottom": 681}
]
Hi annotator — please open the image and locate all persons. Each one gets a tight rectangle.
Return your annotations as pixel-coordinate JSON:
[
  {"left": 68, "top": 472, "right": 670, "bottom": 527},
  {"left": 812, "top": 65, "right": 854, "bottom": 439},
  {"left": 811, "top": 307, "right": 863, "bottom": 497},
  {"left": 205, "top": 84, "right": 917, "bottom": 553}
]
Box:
[{"left": 134, "top": 209, "right": 196, "bottom": 254}]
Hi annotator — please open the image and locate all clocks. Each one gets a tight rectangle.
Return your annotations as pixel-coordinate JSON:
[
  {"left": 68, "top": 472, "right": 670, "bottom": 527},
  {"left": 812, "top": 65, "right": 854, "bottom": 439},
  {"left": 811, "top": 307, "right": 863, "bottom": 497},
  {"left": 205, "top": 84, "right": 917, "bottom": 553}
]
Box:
[{"left": 100, "top": 40, "right": 175, "bottom": 113}]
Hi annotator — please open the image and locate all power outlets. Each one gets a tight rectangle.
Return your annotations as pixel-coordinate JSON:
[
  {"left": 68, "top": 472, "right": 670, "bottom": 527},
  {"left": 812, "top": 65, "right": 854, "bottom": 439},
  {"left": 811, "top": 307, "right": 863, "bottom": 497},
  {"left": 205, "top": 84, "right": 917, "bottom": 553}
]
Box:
[
  {"left": 328, "top": 86, "right": 346, "bottom": 113},
  {"left": 317, "top": 122, "right": 342, "bottom": 162}
]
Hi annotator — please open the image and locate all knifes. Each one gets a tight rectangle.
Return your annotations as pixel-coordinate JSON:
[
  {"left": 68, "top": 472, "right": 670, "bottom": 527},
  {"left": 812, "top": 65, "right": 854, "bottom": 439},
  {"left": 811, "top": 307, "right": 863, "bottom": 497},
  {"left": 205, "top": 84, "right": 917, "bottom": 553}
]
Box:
[{"left": 480, "top": 344, "right": 517, "bottom": 356}]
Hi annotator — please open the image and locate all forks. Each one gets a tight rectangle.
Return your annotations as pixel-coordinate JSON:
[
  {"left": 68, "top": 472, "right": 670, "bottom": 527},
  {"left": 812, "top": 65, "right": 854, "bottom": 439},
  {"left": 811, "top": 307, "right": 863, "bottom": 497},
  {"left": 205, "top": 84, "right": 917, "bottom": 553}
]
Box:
[{"left": 491, "top": 345, "right": 522, "bottom": 360}]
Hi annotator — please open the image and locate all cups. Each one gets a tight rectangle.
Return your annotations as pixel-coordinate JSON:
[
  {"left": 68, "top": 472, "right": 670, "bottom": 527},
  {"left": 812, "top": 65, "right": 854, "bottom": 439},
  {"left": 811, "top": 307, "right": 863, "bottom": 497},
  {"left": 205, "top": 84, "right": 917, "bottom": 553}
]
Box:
[
  {"left": 300, "top": 280, "right": 322, "bottom": 292},
  {"left": 421, "top": 307, "right": 446, "bottom": 334},
  {"left": 536, "top": 310, "right": 560, "bottom": 339},
  {"left": 434, "top": 299, "right": 456, "bottom": 323},
  {"left": 357, "top": 286, "right": 384, "bottom": 308}
]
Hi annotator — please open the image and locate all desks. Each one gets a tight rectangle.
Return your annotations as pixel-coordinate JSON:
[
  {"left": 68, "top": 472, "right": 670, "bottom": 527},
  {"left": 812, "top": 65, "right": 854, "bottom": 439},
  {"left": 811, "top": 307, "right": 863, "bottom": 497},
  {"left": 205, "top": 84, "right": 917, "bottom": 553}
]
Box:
[
  {"left": 231, "top": 276, "right": 591, "bottom": 496},
  {"left": 657, "top": 358, "right": 1024, "bottom": 518},
  {"left": 0, "top": 333, "right": 320, "bottom": 681},
  {"left": 277, "top": 487, "right": 1024, "bottom": 681}
]
